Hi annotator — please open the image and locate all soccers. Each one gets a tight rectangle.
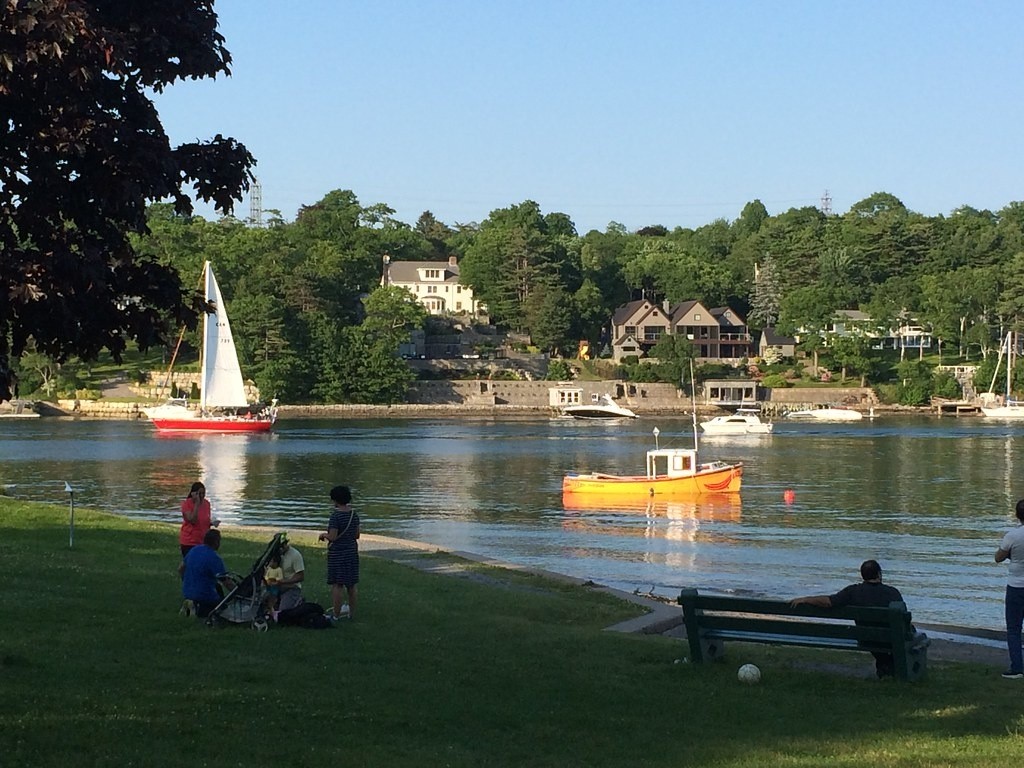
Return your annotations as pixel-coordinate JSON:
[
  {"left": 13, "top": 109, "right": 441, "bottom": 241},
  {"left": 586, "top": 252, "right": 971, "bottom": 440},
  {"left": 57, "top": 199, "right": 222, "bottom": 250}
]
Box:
[{"left": 738, "top": 664, "right": 760, "bottom": 686}]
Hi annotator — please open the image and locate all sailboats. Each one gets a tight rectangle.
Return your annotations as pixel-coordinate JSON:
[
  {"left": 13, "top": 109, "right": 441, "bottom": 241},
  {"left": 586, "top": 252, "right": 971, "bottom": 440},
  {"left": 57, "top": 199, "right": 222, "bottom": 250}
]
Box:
[
  {"left": 139, "top": 260, "right": 280, "bottom": 434},
  {"left": 980, "top": 331, "right": 1024, "bottom": 419}
]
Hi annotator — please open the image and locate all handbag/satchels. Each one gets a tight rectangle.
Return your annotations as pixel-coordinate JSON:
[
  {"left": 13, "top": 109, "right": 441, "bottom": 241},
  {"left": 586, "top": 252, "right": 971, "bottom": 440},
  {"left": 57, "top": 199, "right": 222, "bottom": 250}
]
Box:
[{"left": 278, "top": 603, "right": 331, "bottom": 628}]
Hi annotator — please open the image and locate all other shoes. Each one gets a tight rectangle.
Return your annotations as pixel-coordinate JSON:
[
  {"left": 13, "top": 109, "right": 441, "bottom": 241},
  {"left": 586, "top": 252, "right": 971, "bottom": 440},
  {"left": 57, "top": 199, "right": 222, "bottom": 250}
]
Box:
[
  {"left": 187, "top": 600, "right": 196, "bottom": 617},
  {"left": 324, "top": 613, "right": 340, "bottom": 624},
  {"left": 340, "top": 614, "right": 352, "bottom": 620},
  {"left": 178, "top": 599, "right": 188, "bottom": 616}
]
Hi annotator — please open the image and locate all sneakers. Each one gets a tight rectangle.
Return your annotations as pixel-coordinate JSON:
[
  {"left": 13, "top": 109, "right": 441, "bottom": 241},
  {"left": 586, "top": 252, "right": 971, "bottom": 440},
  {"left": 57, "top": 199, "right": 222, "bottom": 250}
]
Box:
[{"left": 1001, "top": 669, "right": 1023, "bottom": 679}]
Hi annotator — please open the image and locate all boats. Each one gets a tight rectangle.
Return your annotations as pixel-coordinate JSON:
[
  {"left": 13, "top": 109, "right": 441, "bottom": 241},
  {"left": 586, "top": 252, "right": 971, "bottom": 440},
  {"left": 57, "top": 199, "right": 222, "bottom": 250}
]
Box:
[
  {"left": 700, "top": 408, "right": 774, "bottom": 435},
  {"left": 33, "top": 399, "right": 71, "bottom": 417},
  {"left": 785, "top": 408, "right": 864, "bottom": 423},
  {"left": 563, "top": 358, "right": 744, "bottom": 497},
  {"left": 0, "top": 413, "right": 40, "bottom": 420},
  {"left": 562, "top": 393, "right": 641, "bottom": 419}
]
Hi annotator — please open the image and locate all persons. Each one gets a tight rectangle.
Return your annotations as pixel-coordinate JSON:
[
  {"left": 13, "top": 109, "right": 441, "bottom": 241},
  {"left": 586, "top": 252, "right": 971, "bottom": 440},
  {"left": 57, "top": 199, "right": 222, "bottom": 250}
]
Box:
[
  {"left": 264, "top": 533, "right": 306, "bottom": 610},
  {"left": 992, "top": 500, "right": 1024, "bottom": 680},
  {"left": 318, "top": 486, "right": 360, "bottom": 621},
  {"left": 242, "top": 411, "right": 253, "bottom": 420},
  {"left": 785, "top": 560, "right": 926, "bottom": 681},
  {"left": 178, "top": 528, "right": 228, "bottom": 619},
  {"left": 180, "top": 481, "right": 220, "bottom": 560}
]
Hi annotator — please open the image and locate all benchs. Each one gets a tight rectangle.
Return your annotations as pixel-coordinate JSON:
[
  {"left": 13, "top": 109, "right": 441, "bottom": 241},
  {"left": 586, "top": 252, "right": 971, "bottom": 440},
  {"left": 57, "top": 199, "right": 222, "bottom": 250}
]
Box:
[{"left": 674, "top": 586, "right": 932, "bottom": 684}]
